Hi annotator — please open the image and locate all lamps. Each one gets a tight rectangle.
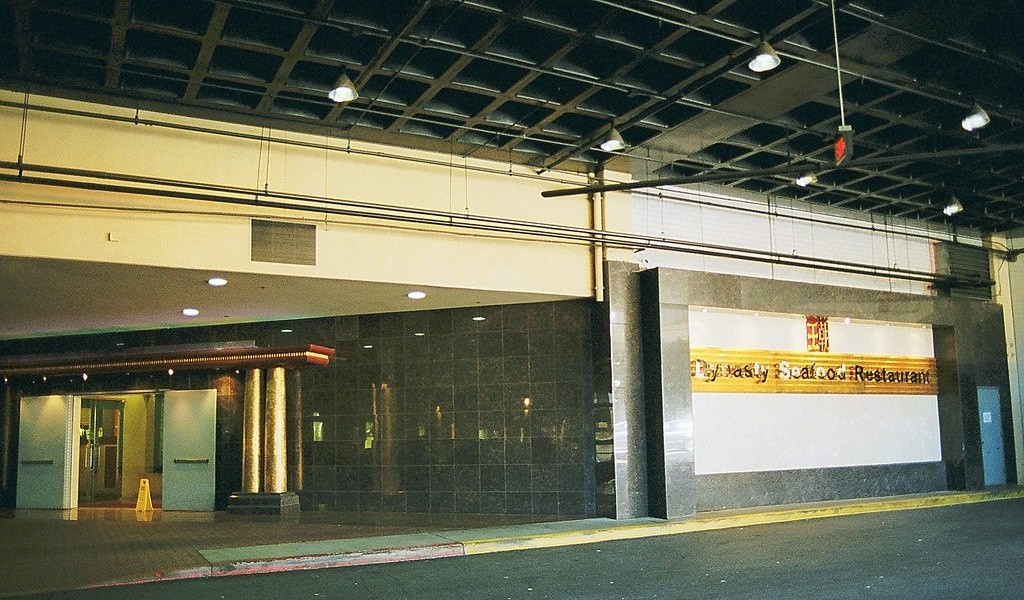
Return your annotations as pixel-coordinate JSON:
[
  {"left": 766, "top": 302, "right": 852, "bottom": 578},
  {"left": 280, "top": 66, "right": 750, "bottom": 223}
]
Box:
[
  {"left": 942, "top": 196, "right": 964, "bottom": 217},
  {"left": 747, "top": 33, "right": 782, "bottom": 73},
  {"left": 796, "top": 171, "right": 818, "bottom": 187},
  {"left": 326, "top": 66, "right": 360, "bottom": 104},
  {"left": 600, "top": 117, "right": 627, "bottom": 152},
  {"left": 960, "top": 99, "right": 992, "bottom": 132}
]
